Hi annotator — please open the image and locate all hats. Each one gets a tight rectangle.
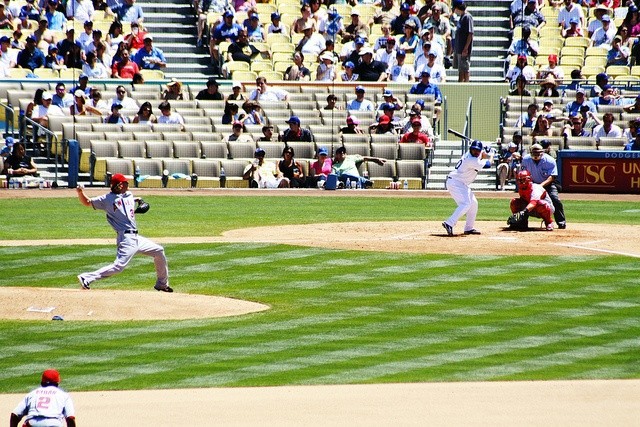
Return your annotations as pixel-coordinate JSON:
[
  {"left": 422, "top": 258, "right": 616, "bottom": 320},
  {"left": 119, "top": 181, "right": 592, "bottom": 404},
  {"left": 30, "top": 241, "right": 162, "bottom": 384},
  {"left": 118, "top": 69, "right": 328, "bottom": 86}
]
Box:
[
  {"left": 271, "top": 12, "right": 280, "bottom": 21},
  {"left": 596, "top": 73, "right": 609, "bottom": 81},
  {"left": 301, "top": 22, "right": 312, "bottom": 31},
  {"left": 143, "top": 34, "right": 153, "bottom": 41},
  {"left": 541, "top": 74, "right": 556, "bottom": 87},
  {"left": 0, "top": 36, "right": 12, "bottom": 44},
  {"left": 90, "top": 86, "right": 98, "bottom": 89},
  {"left": 65, "top": 26, "right": 74, "bottom": 33},
  {"left": 629, "top": 5, "right": 639, "bottom": 12},
  {"left": 110, "top": 174, "right": 130, "bottom": 189},
  {"left": 541, "top": 140, "right": 551, "bottom": 148},
  {"left": 232, "top": 81, "right": 243, "bottom": 90},
  {"left": 318, "top": 148, "right": 329, "bottom": 154},
  {"left": 346, "top": 115, "right": 362, "bottom": 125},
  {"left": 254, "top": 148, "right": 265, "bottom": 157},
  {"left": 421, "top": 69, "right": 431, "bottom": 76},
  {"left": 322, "top": 51, "right": 334, "bottom": 63},
  {"left": 601, "top": 14, "right": 609, "bottom": 22},
  {"left": 350, "top": 10, "right": 360, "bottom": 17},
  {"left": 225, "top": 11, "right": 234, "bottom": 18},
  {"left": 84, "top": 20, "right": 93, "bottom": 27},
  {"left": 285, "top": 116, "right": 300, "bottom": 124},
  {"left": 569, "top": 17, "right": 579, "bottom": 24},
  {"left": 344, "top": 61, "right": 355, "bottom": 70},
  {"left": 422, "top": 42, "right": 430, "bottom": 48},
  {"left": 358, "top": 49, "right": 375, "bottom": 59},
  {"left": 387, "top": 37, "right": 395, "bottom": 41},
  {"left": 419, "top": 29, "right": 431, "bottom": 39},
  {"left": 411, "top": 116, "right": 421, "bottom": 125},
  {"left": 463, "top": 229, "right": 482, "bottom": 234},
  {"left": 80, "top": 73, "right": 88, "bottom": 79},
  {"left": 233, "top": 121, "right": 242, "bottom": 126},
  {"left": 429, "top": 50, "right": 438, "bottom": 57},
  {"left": 48, "top": 44, "right": 59, "bottom": 52},
  {"left": 593, "top": 4, "right": 613, "bottom": 19},
  {"left": 356, "top": 85, "right": 366, "bottom": 94},
  {"left": 543, "top": 99, "right": 553, "bottom": 105},
  {"left": 518, "top": 55, "right": 527, "bottom": 61},
  {"left": 548, "top": 55, "right": 557, "bottom": 62},
  {"left": 5, "top": 137, "right": 14, "bottom": 146},
  {"left": 528, "top": 104, "right": 539, "bottom": 112},
  {"left": 378, "top": 114, "right": 391, "bottom": 126},
  {"left": 26, "top": 35, "right": 37, "bottom": 42},
  {"left": 614, "top": 35, "right": 622, "bottom": 43},
  {"left": 167, "top": 78, "right": 178, "bottom": 87},
  {"left": 507, "top": 142, "right": 517, "bottom": 149},
  {"left": 206, "top": 78, "right": 220, "bottom": 87},
  {"left": 327, "top": 6, "right": 337, "bottom": 16},
  {"left": 400, "top": 3, "right": 411, "bottom": 13},
  {"left": 576, "top": 88, "right": 586, "bottom": 96},
  {"left": 74, "top": 89, "right": 84, "bottom": 98},
  {"left": 49, "top": 0, "right": 58, "bottom": 3},
  {"left": 111, "top": 103, "right": 123, "bottom": 112},
  {"left": 396, "top": 50, "right": 405, "bottom": 56},
  {"left": 355, "top": 37, "right": 364, "bottom": 45},
  {"left": 130, "top": 19, "right": 138, "bottom": 26},
  {"left": 382, "top": 89, "right": 392, "bottom": 97},
  {"left": 417, "top": 99, "right": 424, "bottom": 106},
  {"left": 334, "top": 145, "right": 344, "bottom": 155},
  {"left": 250, "top": 13, "right": 259, "bottom": 20},
  {"left": 383, "top": 103, "right": 395, "bottom": 111},
  {"left": 42, "top": 91, "right": 52, "bottom": 101},
  {"left": 42, "top": 370, "right": 60, "bottom": 384}
]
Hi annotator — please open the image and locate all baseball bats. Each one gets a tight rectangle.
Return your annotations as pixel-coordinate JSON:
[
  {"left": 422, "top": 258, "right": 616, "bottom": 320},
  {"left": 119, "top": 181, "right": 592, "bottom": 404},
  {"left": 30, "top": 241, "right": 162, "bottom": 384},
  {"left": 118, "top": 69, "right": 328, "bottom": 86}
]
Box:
[{"left": 448, "top": 128, "right": 499, "bottom": 154}]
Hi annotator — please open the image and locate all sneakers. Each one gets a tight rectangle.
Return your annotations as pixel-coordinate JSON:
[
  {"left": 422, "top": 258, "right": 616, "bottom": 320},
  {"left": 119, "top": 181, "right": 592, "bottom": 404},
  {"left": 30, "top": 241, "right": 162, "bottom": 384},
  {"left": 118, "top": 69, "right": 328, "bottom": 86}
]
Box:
[
  {"left": 559, "top": 220, "right": 566, "bottom": 229},
  {"left": 76, "top": 274, "right": 89, "bottom": 289},
  {"left": 153, "top": 283, "right": 174, "bottom": 292},
  {"left": 442, "top": 222, "right": 453, "bottom": 237},
  {"left": 546, "top": 222, "right": 555, "bottom": 231}
]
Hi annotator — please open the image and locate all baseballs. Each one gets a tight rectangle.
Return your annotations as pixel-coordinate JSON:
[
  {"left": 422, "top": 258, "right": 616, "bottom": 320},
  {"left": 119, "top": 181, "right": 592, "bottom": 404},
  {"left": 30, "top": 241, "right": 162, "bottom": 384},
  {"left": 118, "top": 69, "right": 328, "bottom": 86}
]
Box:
[
  {"left": 164, "top": 169, "right": 169, "bottom": 176},
  {"left": 78, "top": 184, "right": 84, "bottom": 190}
]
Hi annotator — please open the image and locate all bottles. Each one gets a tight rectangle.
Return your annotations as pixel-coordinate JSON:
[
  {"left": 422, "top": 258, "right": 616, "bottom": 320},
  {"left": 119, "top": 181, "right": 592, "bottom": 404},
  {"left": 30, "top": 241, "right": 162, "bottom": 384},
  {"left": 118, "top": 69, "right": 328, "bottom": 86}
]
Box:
[
  {"left": 357, "top": 178, "right": 361, "bottom": 188},
  {"left": 190, "top": 172, "right": 198, "bottom": 187},
  {"left": 345, "top": 178, "right": 350, "bottom": 188},
  {"left": 161, "top": 169, "right": 169, "bottom": 187},
  {"left": 39, "top": 176, "right": 43, "bottom": 189},
  {"left": 351, "top": 181, "right": 356, "bottom": 189},
  {"left": 9, "top": 178, "right": 13, "bottom": 190},
  {"left": 14, "top": 178, "right": 19, "bottom": 189},
  {"left": 219, "top": 166, "right": 226, "bottom": 189},
  {"left": 403, "top": 179, "right": 409, "bottom": 190},
  {"left": 134, "top": 165, "right": 141, "bottom": 188},
  {"left": 22, "top": 178, "right": 27, "bottom": 189}
]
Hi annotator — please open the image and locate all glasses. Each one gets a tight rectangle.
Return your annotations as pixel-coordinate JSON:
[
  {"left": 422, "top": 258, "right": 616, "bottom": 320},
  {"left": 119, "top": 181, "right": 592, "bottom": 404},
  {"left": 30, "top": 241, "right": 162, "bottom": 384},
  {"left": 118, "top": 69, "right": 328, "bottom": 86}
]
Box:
[
  {"left": 572, "top": 122, "right": 582, "bottom": 125},
  {"left": 143, "top": 108, "right": 150, "bottom": 112},
  {"left": 257, "top": 82, "right": 266, "bottom": 85},
  {"left": 285, "top": 150, "right": 291, "bottom": 154},
  {"left": 621, "top": 30, "right": 628, "bottom": 32},
  {"left": 117, "top": 91, "right": 126, "bottom": 94}
]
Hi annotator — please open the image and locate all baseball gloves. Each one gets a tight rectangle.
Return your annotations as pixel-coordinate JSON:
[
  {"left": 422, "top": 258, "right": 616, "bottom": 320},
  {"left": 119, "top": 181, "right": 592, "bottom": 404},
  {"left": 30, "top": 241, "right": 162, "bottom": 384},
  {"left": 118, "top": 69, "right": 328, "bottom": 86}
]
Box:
[
  {"left": 135, "top": 200, "right": 151, "bottom": 214},
  {"left": 508, "top": 212, "right": 528, "bottom": 229}
]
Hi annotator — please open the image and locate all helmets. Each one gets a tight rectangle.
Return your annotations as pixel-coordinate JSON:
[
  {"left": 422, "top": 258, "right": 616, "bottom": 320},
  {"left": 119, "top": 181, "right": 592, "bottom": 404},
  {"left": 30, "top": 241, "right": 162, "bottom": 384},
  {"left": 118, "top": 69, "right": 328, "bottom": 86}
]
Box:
[
  {"left": 516, "top": 170, "right": 531, "bottom": 190},
  {"left": 469, "top": 140, "right": 483, "bottom": 152},
  {"left": 527, "top": 144, "right": 548, "bottom": 161}
]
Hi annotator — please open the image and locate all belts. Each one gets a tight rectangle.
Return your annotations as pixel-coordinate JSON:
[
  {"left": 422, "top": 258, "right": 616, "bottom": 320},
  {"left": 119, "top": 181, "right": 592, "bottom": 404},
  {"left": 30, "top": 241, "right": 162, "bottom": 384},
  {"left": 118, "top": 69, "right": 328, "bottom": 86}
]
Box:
[{"left": 125, "top": 230, "right": 138, "bottom": 234}]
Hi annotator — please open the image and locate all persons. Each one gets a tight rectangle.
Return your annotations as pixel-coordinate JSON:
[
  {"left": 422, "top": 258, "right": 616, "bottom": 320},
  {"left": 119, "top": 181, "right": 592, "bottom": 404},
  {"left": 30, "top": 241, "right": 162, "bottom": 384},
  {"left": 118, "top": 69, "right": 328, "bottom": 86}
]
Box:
[
  {"left": 132, "top": 100, "right": 157, "bottom": 125},
  {"left": 87, "top": 83, "right": 100, "bottom": 98},
  {"left": 242, "top": 146, "right": 290, "bottom": 188},
  {"left": 26, "top": 87, "right": 47, "bottom": 125},
  {"left": 85, "top": 92, "right": 109, "bottom": 109},
  {"left": 541, "top": 138, "right": 557, "bottom": 160},
  {"left": 0, "top": 136, "right": 14, "bottom": 157},
  {"left": 329, "top": 143, "right": 387, "bottom": 189},
  {"left": 68, "top": 88, "right": 103, "bottom": 117},
  {"left": 495, "top": 129, "right": 527, "bottom": 191},
  {"left": 623, "top": 134, "right": 640, "bottom": 152},
  {"left": 399, "top": 118, "right": 430, "bottom": 147},
  {"left": 450, "top": 0, "right": 474, "bottom": 82},
  {"left": 391, "top": 103, "right": 433, "bottom": 135},
  {"left": 69, "top": 73, "right": 91, "bottom": 94},
  {"left": 0, "top": 0, "right": 167, "bottom": 78},
  {"left": 2, "top": 142, "right": 40, "bottom": 188},
  {"left": 31, "top": 91, "right": 64, "bottom": 130},
  {"left": 162, "top": 77, "right": 184, "bottom": 100},
  {"left": 338, "top": 115, "right": 365, "bottom": 135},
  {"left": 256, "top": 125, "right": 279, "bottom": 142},
  {"left": 308, "top": 145, "right": 337, "bottom": 190},
  {"left": 108, "top": 85, "right": 141, "bottom": 110},
  {"left": 51, "top": 82, "right": 73, "bottom": 108},
  {"left": 519, "top": 143, "right": 566, "bottom": 229},
  {"left": 323, "top": 95, "right": 344, "bottom": 111},
  {"left": 229, "top": 80, "right": 248, "bottom": 100},
  {"left": 373, "top": 114, "right": 398, "bottom": 135},
  {"left": 75, "top": 172, "right": 175, "bottom": 293},
  {"left": 248, "top": 76, "right": 291, "bottom": 103},
  {"left": 401, "top": 110, "right": 429, "bottom": 134},
  {"left": 276, "top": 146, "right": 306, "bottom": 188},
  {"left": 408, "top": 68, "right": 442, "bottom": 114},
  {"left": 506, "top": 169, "right": 556, "bottom": 231},
  {"left": 441, "top": 139, "right": 496, "bottom": 236},
  {"left": 346, "top": 85, "right": 374, "bottom": 112},
  {"left": 505, "top": 0, "right": 640, "bottom": 137},
  {"left": 9, "top": 368, "right": 76, "bottom": 427},
  {"left": 416, "top": 99, "right": 426, "bottom": 112},
  {"left": 156, "top": 100, "right": 184, "bottom": 124},
  {"left": 220, "top": 120, "right": 255, "bottom": 143},
  {"left": 130, "top": 72, "right": 145, "bottom": 92},
  {"left": 376, "top": 89, "right": 404, "bottom": 111},
  {"left": 281, "top": 115, "right": 314, "bottom": 142},
  {"left": 222, "top": 100, "right": 265, "bottom": 125},
  {"left": 376, "top": 104, "right": 399, "bottom": 122},
  {"left": 103, "top": 102, "right": 129, "bottom": 125},
  {"left": 194, "top": 78, "right": 225, "bottom": 101},
  {"left": 190, "top": 0, "right": 453, "bottom": 81}
]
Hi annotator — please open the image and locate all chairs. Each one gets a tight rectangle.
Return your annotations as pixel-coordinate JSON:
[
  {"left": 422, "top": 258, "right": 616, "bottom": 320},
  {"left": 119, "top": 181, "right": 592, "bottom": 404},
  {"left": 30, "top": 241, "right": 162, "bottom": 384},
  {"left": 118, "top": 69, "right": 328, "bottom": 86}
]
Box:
[
  {"left": 502, "top": 94, "right": 640, "bottom": 161},
  {"left": 0, "top": 1, "right": 165, "bottom": 78},
  {"left": 507, "top": 0, "right": 640, "bottom": 84},
  {"left": 203, "top": 0, "right": 416, "bottom": 81}
]
